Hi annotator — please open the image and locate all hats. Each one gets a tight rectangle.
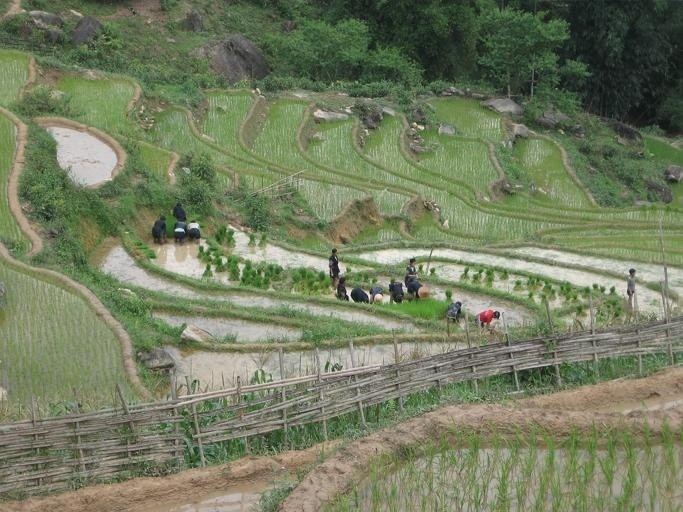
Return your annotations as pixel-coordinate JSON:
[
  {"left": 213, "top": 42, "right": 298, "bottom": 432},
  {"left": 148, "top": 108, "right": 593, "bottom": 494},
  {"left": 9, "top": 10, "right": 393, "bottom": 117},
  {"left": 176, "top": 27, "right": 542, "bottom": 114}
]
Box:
[
  {"left": 417, "top": 286, "right": 430, "bottom": 300},
  {"left": 373, "top": 293, "right": 383, "bottom": 305}
]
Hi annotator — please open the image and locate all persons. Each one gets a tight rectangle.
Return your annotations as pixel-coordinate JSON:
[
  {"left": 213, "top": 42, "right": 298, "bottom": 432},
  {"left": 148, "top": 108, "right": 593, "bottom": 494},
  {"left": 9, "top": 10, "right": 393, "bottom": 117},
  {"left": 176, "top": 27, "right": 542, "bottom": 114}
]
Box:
[
  {"left": 446, "top": 301, "right": 462, "bottom": 322},
  {"left": 474, "top": 309, "right": 499, "bottom": 327},
  {"left": 626, "top": 268, "right": 635, "bottom": 308},
  {"left": 404, "top": 258, "right": 421, "bottom": 287},
  {"left": 173, "top": 202, "right": 186, "bottom": 221},
  {"left": 406, "top": 280, "right": 429, "bottom": 301},
  {"left": 350, "top": 288, "right": 368, "bottom": 303},
  {"left": 173, "top": 218, "right": 186, "bottom": 244},
  {"left": 335, "top": 276, "right": 348, "bottom": 301},
  {"left": 328, "top": 248, "right": 339, "bottom": 290},
  {"left": 368, "top": 287, "right": 384, "bottom": 304},
  {"left": 187, "top": 220, "right": 201, "bottom": 244},
  {"left": 389, "top": 278, "right": 404, "bottom": 303},
  {"left": 151, "top": 215, "right": 167, "bottom": 245}
]
